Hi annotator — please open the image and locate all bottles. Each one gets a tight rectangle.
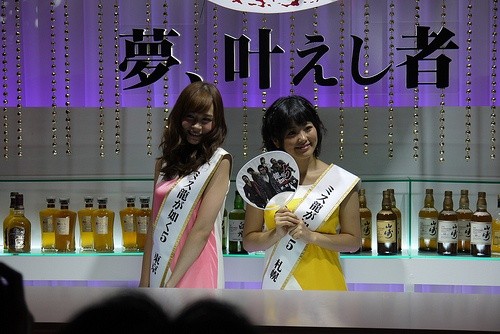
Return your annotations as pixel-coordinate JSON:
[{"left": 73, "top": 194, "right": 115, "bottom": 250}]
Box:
[
  {"left": 39, "top": 197, "right": 57, "bottom": 252},
  {"left": 470, "top": 192, "right": 492, "bottom": 257},
  {"left": 376, "top": 191, "right": 397, "bottom": 255},
  {"left": 119, "top": 196, "right": 138, "bottom": 251},
  {"left": 358, "top": 188, "right": 372, "bottom": 252},
  {"left": 437, "top": 191, "right": 458, "bottom": 256},
  {"left": 91, "top": 197, "right": 115, "bottom": 252},
  {"left": 222, "top": 208, "right": 228, "bottom": 254},
  {"left": 229, "top": 190, "right": 247, "bottom": 254},
  {"left": 132, "top": 196, "right": 151, "bottom": 252},
  {"left": 53, "top": 197, "right": 76, "bottom": 253},
  {"left": 418, "top": 189, "right": 438, "bottom": 252},
  {"left": 2, "top": 192, "right": 19, "bottom": 253},
  {"left": 387, "top": 188, "right": 401, "bottom": 253},
  {"left": 492, "top": 194, "right": 500, "bottom": 257},
  {"left": 7, "top": 194, "right": 32, "bottom": 253},
  {"left": 455, "top": 190, "right": 473, "bottom": 254},
  {"left": 77, "top": 196, "right": 95, "bottom": 251}
]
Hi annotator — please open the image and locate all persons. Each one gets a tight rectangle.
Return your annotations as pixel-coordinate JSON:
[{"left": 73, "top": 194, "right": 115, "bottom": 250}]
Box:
[
  {"left": 242, "top": 156, "right": 298, "bottom": 208},
  {"left": 242, "top": 95, "right": 361, "bottom": 290},
  {"left": 139, "top": 81, "right": 233, "bottom": 288}
]
